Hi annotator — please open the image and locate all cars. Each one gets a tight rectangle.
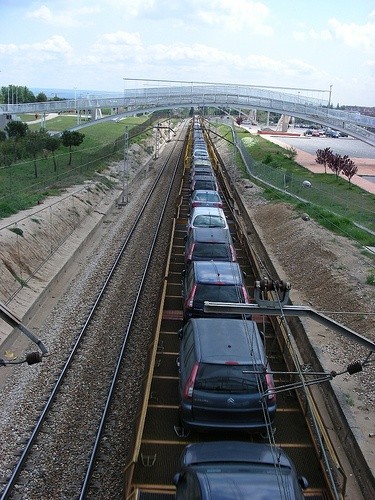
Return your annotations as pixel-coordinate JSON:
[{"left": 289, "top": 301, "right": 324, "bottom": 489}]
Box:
[
  {"left": 288, "top": 123, "right": 348, "bottom": 139},
  {"left": 186, "top": 207, "right": 229, "bottom": 236},
  {"left": 181, "top": 260, "right": 253, "bottom": 324},
  {"left": 176, "top": 318, "right": 278, "bottom": 431},
  {"left": 187, "top": 189, "right": 223, "bottom": 214},
  {"left": 189, "top": 114, "right": 218, "bottom": 196},
  {"left": 183, "top": 227, "right": 236, "bottom": 265},
  {"left": 173, "top": 441, "right": 310, "bottom": 500}
]
